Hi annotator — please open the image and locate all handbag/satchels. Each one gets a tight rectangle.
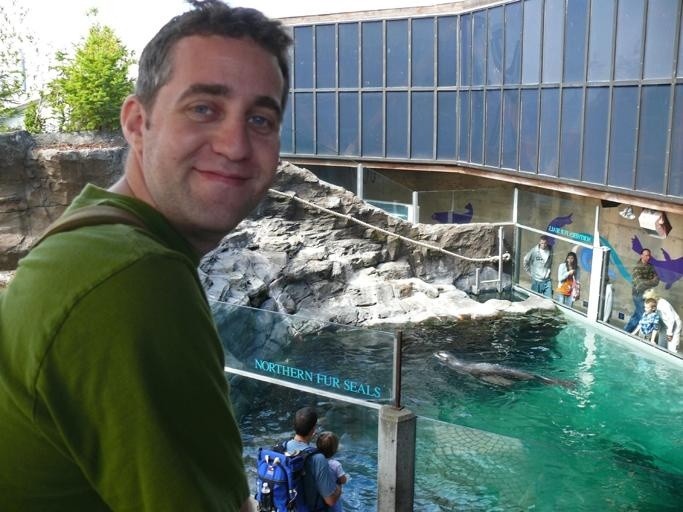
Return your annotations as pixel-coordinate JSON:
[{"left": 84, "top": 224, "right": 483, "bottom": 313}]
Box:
[{"left": 556, "top": 277, "right": 581, "bottom": 302}]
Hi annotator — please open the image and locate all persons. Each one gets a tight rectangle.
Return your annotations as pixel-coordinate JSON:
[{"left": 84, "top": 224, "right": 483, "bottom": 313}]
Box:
[
  {"left": 557, "top": 251, "right": 580, "bottom": 308},
  {"left": 313, "top": 431, "right": 348, "bottom": 486},
  {"left": 269, "top": 405, "right": 342, "bottom": 511},
  {"left": 622, "top": 247, "right": 660, "bottom": 332},
  {"left": 0, "top": 1, "right": 297, "bottom": 511},
  {"left": 630, "top": 298, "right": 658, "bottom": 346},
  {"left": 521, "top": 235, "right": 554, "bottom": 298},
  {"left": 641, "top": 288, "right": 682, "bottom": 354}
]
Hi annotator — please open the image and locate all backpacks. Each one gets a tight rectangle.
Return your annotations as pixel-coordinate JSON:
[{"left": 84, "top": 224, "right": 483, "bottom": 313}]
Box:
[{"left": 255, "top": 437, "right": 324, "bottom": 512}]
